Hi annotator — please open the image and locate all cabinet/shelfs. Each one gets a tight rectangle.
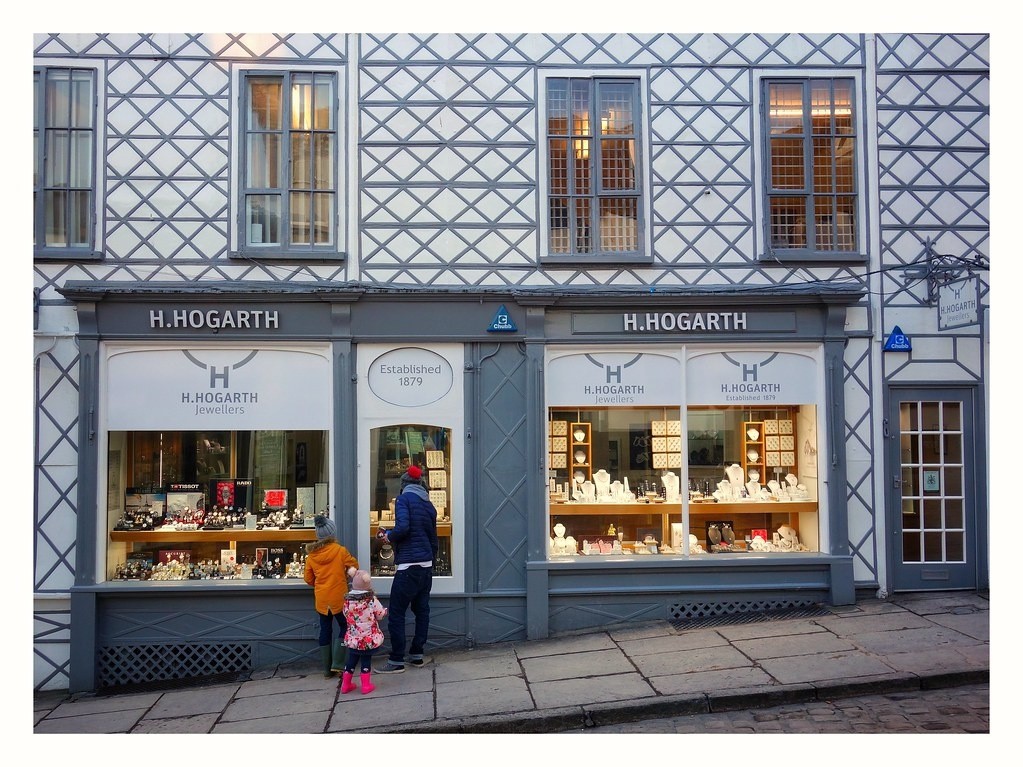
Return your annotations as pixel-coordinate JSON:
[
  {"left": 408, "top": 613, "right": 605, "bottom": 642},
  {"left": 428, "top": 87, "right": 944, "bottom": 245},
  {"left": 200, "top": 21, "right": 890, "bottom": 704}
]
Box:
[
  {"left": 550, "top": 498, "right": 817, "bottom": 554},
  {"left": 110, "top": 518, "right": 452, "bottom": 578},
  {"left": 740, "top": 421, "right": 767, "bottom": 486},
  {"left": 570, "top": 422, "right": 593, "bottom": 490}
]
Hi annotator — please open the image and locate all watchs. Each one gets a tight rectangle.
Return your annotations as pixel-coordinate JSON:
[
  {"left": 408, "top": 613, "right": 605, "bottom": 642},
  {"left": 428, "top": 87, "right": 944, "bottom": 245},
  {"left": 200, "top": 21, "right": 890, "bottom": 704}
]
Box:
[
  {"left": 118, "top": 505, "right": 248, "bottom": 529},
  {"left": 116, "top": 552, "right": 242, "bottom": 581},
  {"left": 222, "top": 486, "right": 229, "bottom": 503}
]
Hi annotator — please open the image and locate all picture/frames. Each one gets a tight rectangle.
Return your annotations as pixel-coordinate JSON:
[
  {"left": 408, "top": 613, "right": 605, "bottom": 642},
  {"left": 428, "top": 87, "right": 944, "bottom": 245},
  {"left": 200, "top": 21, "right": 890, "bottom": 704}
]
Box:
[
  {"left": 923, "top": 470, "right": 940, "bottom": 493},
  {"left": 931, "top": 424, "right": 948, "bottom": 455}
]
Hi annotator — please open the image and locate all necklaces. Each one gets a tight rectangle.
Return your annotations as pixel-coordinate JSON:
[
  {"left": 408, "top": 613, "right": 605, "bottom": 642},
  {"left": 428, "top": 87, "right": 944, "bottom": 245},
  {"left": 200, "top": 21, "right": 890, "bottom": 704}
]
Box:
[
  {"left": 689, "top": 462, "right": 811, "bottom": 501},
  {"left": 550, "top": 524, "right": 578, "bottom": 556},
  {"left": 707, "top": 522, "right": 804, "bottom": 551},
  {"left": 573, "top": 468, "right": 680, "bottom": 505},
  {"left": 574, "top": 428, "right": 586, "bottom": 463},
  {"left": 378, "top": 548, "right": 449, "bottom": 574},
  {"left": 746, "top": 428, "right": 760, "bottom": 462}
]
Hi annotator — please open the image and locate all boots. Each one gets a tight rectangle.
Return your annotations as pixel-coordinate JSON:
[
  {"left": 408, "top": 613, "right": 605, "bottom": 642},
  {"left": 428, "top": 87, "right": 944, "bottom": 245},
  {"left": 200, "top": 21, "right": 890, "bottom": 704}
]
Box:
[
  {"left": 331, "top": 638, "right": 346, "bottom": 672},
  {"left": 341, "top": 673, "right": 357, "bottom": 694},
  {"left": 359, "top": 672, "right": 375, "bottom": 694},
  {"left": 319, "top": 645, "right": 342, "bottom": 680}
]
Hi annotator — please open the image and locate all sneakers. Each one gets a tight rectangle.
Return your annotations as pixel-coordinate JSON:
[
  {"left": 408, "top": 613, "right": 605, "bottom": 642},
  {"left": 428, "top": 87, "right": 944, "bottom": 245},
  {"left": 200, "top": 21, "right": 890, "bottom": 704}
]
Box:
[
  {"left": 402, "top": 656, "right": 425, "bottom": 668},
  {"left": 373, "top": 663, "right": 405, "bottom": 675}
]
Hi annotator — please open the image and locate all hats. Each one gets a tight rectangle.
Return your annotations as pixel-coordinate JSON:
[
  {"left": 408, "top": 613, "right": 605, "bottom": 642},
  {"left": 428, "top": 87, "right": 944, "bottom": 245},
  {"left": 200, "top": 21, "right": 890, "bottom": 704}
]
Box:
[
  {"left": 347, "top": 566, "right": 373, "bottom": 591},
  {"left": 314, "top": 515, "right": 336, "bottom": 541},
  {"left": 400, "top": 466, "right": 424, "bottom": 485}
]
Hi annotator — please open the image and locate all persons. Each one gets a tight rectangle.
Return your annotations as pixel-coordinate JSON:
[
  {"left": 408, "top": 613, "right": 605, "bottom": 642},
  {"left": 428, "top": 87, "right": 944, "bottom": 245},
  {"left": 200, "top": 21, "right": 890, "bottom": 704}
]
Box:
[
  {"left": 341, "top": 566, "right": 387, "bottom": 695},
  {"left": 402, "top": 456, "right": 409, "bottom": 468},
  {"left": 304, "top": 515, "right": 360, "bottom": 678},
  {"left": 373, "top": 466, "right": 438, "bottom": 674}
]
